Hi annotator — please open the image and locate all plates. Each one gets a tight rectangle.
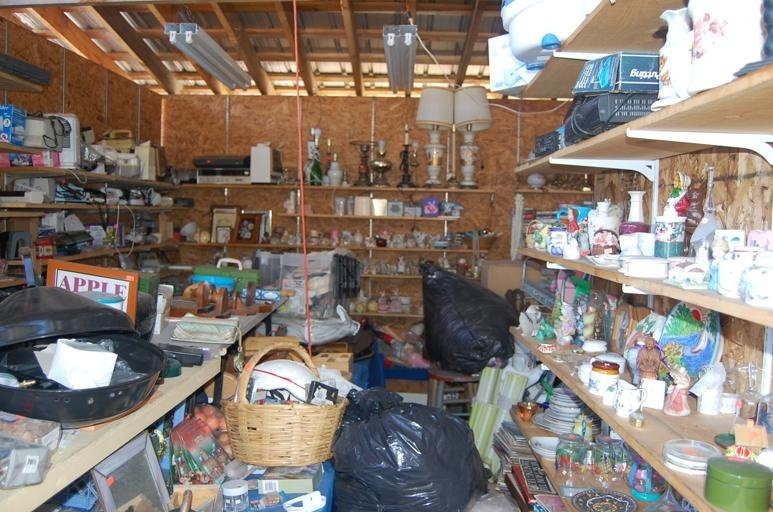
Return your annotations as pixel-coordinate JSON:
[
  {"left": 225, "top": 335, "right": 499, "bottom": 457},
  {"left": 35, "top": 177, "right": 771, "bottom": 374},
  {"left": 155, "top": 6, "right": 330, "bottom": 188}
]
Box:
[
  {"left": 531, "top": 387, "right": 601, "bottom": 436},
  {"left": 527, "top": 436, "right": 560, "bottom": 461}
]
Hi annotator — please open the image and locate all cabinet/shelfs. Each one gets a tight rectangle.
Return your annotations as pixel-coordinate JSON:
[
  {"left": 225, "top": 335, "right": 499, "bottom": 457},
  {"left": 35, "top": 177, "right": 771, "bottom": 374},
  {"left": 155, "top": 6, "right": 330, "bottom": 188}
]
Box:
[
  {"left": 175, "top": 182, "right": 503, "bottom": 318},
  {"left": 1, "top": 142, "right": 172, "bottom": 290},
  {"left": 508, "top": 0, "right": 772, "bottom": 511},
  {"left": 1, "top": 287, "right": 290, "bottom": 512}
]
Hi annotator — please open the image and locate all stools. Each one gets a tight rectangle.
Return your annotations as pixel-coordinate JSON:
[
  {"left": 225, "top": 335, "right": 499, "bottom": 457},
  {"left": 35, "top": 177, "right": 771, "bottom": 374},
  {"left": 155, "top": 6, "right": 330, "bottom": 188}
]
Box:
[{"left": 428, "top": 368, "right": 477, "bottom": 418}]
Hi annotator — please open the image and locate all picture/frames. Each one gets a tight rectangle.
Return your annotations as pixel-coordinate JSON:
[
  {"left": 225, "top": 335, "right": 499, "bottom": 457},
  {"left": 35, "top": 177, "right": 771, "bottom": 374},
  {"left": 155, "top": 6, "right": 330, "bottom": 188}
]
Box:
[
  {"left": 259, "top": 209, "right": 271, "bottom": 240},
  {"left": 209, "top": 206, "right": 239, "bottom": 244},
  {"left": 259, "top": 214, "right": 265, "bottom": 244},
  {"left": 91, "top": 432, "right": 173, "bottom": 512},
  {"left": 231, "top": 212, "right": 261, "bottom": 244}
]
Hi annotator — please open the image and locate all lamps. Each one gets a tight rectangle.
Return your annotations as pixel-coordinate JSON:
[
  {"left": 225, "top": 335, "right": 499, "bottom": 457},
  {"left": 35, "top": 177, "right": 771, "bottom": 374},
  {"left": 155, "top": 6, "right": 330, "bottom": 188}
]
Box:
[
  {"left": 309, "top": 127, "right": 323, "bottom": 187},
  {"left": 370, "top": 140, "right": 393, "bottom": 189},
  {"left": 414, "top": 88, "right": 454, "bottom": 189},
  {"left": 181, "top": 24, "right": 252, "bottom": 92},
  {"left": 383, "top": 24, "right": 401, "bottom": 95},
  {"left": 455, "top": 84, "right": 492, "bottom": 188},
  {"left": 400, "top": 24, "right": 417, "bottom": 100},
  {"left": 404, "top": 122, "right": 410, "bottom": 145},
  {"left": 164, "top": 23, "right": 239, "bottom": 91}
]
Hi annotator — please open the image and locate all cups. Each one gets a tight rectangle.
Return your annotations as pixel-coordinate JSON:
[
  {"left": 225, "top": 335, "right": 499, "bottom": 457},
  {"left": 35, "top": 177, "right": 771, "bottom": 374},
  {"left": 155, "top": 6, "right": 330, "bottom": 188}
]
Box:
[
  {"left": 613, "top": 378, "right": 647, "bottom": 419},
  {"left": 516, "top": 401, "right": 539, "bottom": 423}
]
